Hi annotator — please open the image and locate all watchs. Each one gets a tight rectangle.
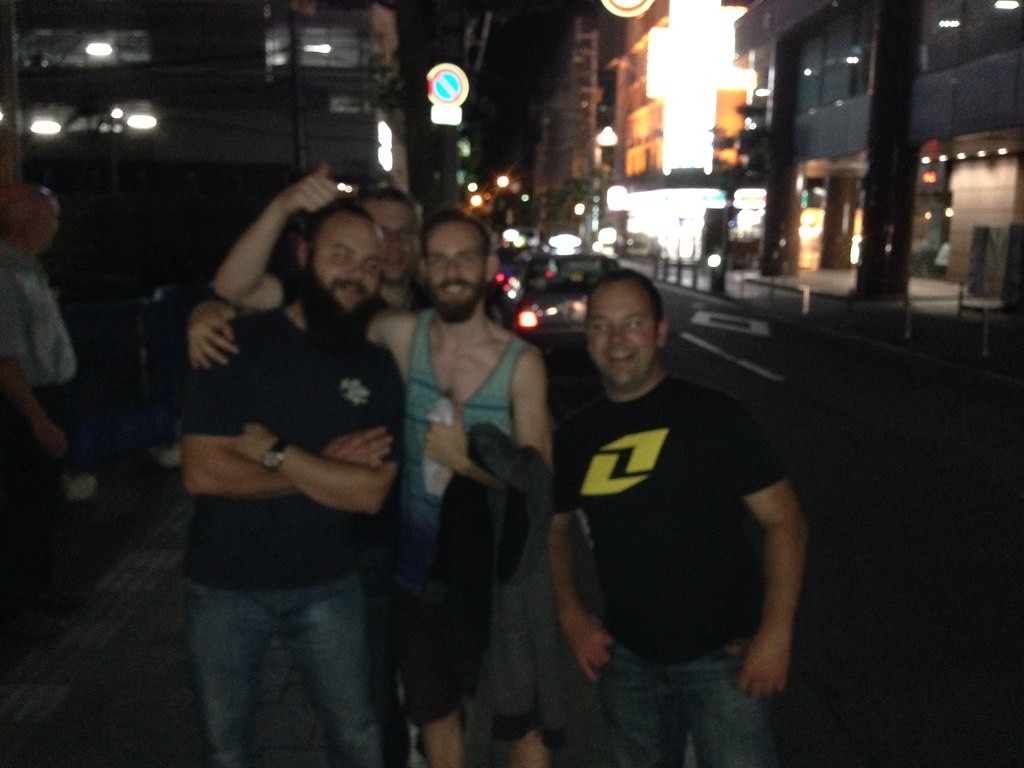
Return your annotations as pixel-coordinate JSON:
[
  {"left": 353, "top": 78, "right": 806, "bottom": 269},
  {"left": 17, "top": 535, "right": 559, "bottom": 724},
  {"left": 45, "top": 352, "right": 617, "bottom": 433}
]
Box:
[{"left": 260, "top": 439, "right": 289, "bottom": 471}]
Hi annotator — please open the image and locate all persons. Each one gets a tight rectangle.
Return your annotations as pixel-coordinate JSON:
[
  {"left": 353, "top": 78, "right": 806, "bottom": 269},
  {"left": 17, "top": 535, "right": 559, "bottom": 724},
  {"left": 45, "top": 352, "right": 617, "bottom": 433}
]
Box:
[
  {"left": 181, "top": 203, "right": 403, "bottom": 768},
  {"left": 0, "top": 184, "right": 75, "bottom": 637},
  {"left": 546, "top": 268, "right": 809, "bottom": 768},
  {"left": 187, "top": 210, "right": 552, "bottom": 768},
  {"left": 214, "top": 166, "right": 426, "bottom": 768}
]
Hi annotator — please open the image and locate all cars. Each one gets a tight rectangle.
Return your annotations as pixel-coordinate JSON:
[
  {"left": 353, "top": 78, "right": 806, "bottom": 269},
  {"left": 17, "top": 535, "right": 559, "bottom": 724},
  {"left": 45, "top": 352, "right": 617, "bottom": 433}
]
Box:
[{"left": 492, "top": 244, "right": 620, "bottom": 337}]
